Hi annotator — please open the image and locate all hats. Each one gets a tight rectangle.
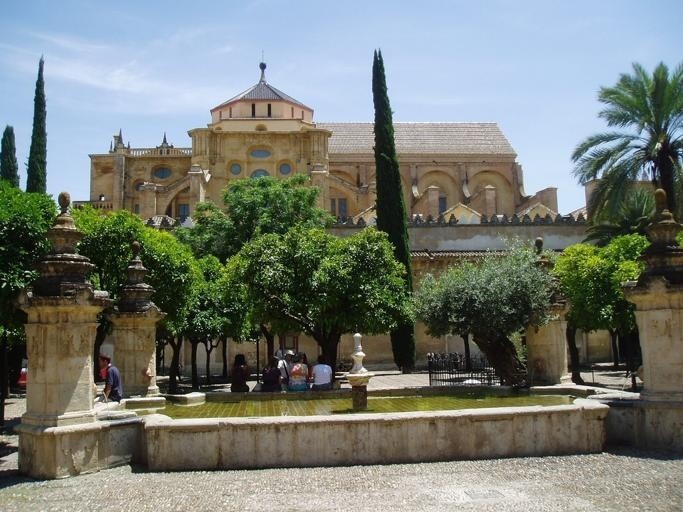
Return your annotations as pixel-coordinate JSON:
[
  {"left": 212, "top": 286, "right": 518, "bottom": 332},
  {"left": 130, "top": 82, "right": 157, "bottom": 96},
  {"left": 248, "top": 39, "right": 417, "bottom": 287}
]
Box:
[{"left": 284, "top": 350, "right": 295, "bottom": 356}]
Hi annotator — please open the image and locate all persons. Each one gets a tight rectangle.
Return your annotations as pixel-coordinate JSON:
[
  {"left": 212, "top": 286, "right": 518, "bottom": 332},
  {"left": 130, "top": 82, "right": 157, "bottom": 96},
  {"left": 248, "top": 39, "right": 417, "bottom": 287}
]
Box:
[
  {"left": 96, "top": 353, "right": 122, "bottom": 402},
  {"left": 229, "top": 349, "right": 334, "bottom": 394}
]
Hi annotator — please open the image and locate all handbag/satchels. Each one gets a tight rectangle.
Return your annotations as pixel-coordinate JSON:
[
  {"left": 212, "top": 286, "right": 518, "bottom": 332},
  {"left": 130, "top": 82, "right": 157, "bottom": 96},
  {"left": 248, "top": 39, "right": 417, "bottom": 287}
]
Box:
[{"left": 253, "top": 383, "right": 262, "bottom": 392}]
